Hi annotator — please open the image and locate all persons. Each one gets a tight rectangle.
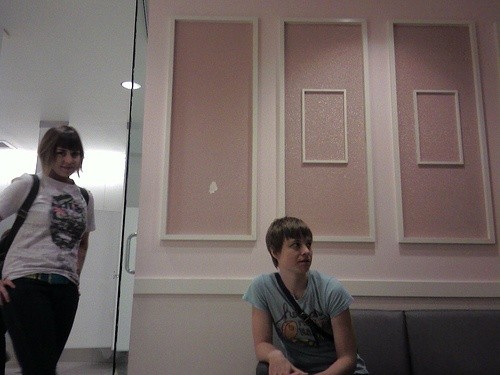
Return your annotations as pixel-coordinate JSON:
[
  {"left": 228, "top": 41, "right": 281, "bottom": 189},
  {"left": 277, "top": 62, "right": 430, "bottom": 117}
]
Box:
[
  {"left": 0, "top": 125, "right": 96, "bottom": 375},
  {"left": 242, "top": 217, "right": 370, "bottom": 375}
]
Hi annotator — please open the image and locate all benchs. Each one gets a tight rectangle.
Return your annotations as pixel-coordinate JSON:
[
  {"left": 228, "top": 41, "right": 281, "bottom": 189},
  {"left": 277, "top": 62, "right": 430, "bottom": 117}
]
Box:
[{"left": 256, "top": 311, "right": 500, "bottom": 375}]
